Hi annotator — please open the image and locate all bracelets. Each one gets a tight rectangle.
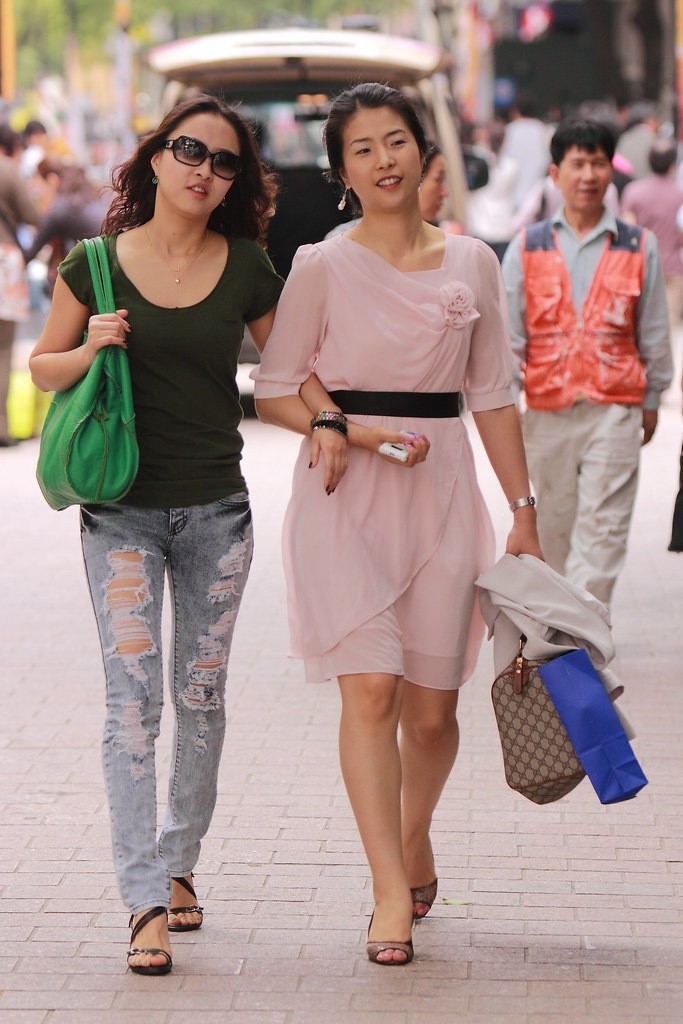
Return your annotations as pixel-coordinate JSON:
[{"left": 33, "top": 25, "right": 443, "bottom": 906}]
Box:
[
  {"left": 310, "top": 411, "right": 348, "bottom": 438},
  {"left": 508, "top": 496, "right": 535, "bottom": 512}
]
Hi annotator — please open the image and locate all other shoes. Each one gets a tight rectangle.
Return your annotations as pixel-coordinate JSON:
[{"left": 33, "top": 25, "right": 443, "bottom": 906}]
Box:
[
  {"left": 367, "top": 908, "right": 412, "bottom": 966},
  {"left": 411, "top": 880, "right": 437, "bottom": 920}
]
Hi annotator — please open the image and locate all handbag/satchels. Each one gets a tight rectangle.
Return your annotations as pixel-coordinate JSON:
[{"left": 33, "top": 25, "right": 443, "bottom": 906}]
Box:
[
  {"left": 36, "top": 237, "right": 139, "bottom": 510},
  {"left": 491, "top": 629, "right": 647, "bottom": 803}
]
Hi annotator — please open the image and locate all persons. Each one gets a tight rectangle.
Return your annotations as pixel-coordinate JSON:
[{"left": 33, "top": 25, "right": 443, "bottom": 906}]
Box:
[{"left": 0, "top": 82, "right": 683, "bottom": 974}]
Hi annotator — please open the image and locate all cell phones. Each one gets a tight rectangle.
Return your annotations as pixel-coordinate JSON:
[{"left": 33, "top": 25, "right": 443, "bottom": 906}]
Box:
[{"left": 379, "top": 430, "right": 416, "bottom": 462}]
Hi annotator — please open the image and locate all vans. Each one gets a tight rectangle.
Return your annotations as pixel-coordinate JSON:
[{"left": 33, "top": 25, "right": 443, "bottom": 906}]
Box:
[{"left": 147, "top": 23, "right": 492, "bottom": 410}]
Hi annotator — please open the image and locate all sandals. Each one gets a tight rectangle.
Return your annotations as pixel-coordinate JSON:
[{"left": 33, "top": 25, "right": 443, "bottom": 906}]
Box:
[
  {"left": 126, "top": 906, "right": 173, "bottom": 974},
  {"left": 167, "top": 873, "right": 203, "bottom": 931}
]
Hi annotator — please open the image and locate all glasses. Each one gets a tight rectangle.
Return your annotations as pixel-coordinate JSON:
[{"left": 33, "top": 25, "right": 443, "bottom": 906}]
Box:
[{"left": 162, "top": 135, "right": 243, "bottom": 180}]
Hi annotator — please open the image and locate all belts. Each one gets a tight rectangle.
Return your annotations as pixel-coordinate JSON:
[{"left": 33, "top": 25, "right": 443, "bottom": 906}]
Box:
[{"left": 329, "top": 388, "right": 463, "bottom": 419}]
